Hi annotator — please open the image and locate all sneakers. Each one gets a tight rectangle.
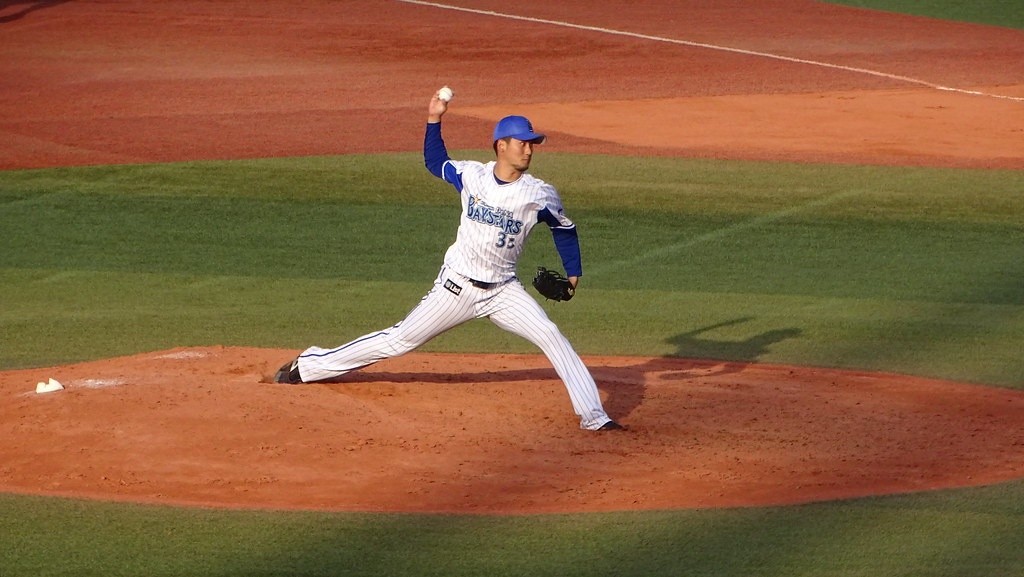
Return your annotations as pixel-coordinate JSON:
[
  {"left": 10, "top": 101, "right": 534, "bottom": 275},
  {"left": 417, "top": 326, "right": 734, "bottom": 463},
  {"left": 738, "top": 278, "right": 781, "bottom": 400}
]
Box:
[
  {"left": 272, "top": 355, "right": 303, "bottom": 384},
  {"left": 597, "top": 421, "right": 623, "bottom": 431}
]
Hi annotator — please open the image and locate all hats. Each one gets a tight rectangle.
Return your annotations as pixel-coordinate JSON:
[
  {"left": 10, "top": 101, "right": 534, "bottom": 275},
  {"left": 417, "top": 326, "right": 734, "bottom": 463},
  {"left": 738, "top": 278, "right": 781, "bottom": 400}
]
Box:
[{"left": 492, "top": 115, "right": 545, "bottom": 144}]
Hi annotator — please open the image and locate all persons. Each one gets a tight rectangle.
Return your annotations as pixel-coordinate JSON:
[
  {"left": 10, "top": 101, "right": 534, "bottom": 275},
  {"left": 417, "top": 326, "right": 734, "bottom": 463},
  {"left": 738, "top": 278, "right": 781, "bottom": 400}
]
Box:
[{"left": 272, "top": 85, "right": 628, "bottom": 433}]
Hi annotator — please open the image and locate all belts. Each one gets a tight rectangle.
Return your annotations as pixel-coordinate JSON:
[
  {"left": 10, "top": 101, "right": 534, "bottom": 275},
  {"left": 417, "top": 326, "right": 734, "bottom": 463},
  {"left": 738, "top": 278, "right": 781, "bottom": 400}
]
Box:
[{"left": 469, "top": 276, "right": 516, "bottom": 289}]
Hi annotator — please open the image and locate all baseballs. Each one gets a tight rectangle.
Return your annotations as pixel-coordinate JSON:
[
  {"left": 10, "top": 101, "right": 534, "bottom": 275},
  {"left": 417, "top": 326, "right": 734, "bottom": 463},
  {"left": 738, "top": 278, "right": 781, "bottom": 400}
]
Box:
[{"left": 438, "top": 87, "right": 452, "bottom": 102}]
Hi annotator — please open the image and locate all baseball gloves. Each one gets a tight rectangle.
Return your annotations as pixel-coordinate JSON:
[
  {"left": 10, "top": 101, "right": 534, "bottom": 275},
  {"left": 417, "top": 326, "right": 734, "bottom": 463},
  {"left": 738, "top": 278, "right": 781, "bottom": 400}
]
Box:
[{"left": 530, "top": 267, "right": 576, "bottom": 303}]
